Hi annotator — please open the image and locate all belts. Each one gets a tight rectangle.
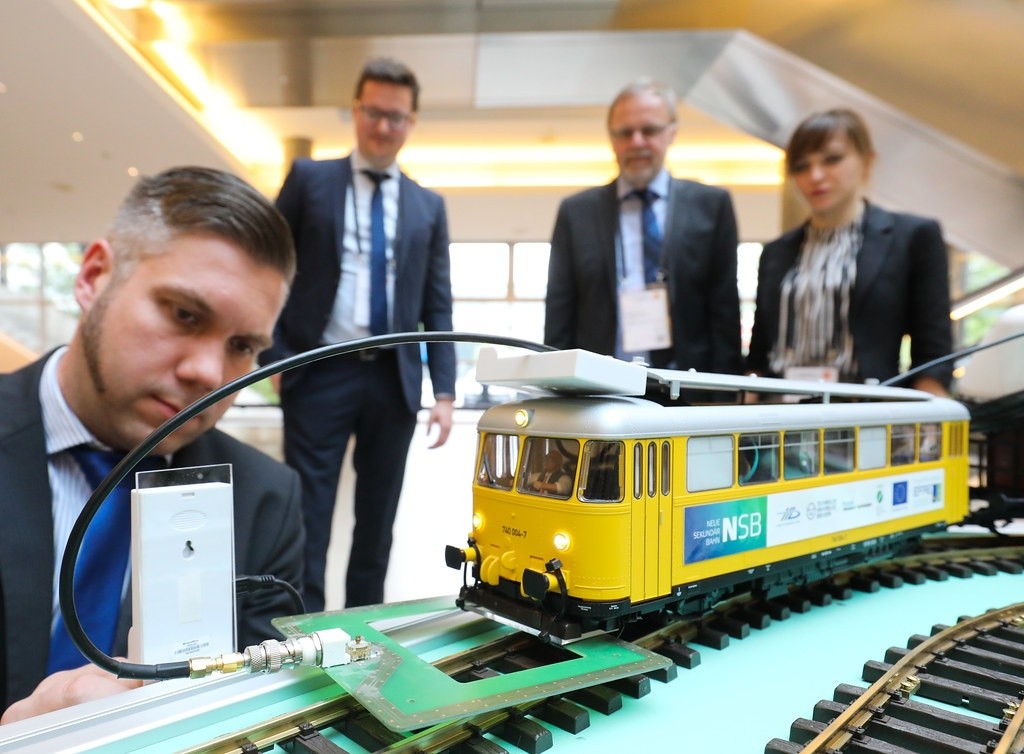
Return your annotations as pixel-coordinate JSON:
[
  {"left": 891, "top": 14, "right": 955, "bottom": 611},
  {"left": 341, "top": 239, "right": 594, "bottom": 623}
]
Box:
[{"left": 319, "top": 343, "right": 396, "bottom": 362}]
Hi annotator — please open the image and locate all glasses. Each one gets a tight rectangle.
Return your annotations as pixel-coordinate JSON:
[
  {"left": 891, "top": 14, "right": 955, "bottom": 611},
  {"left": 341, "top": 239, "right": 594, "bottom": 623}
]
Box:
[
  {"left": 362, "top": 105, "right": 416, "bottom": 127},
  {"left": 613, "top": 120, "right": 675, "bottom": 140}
]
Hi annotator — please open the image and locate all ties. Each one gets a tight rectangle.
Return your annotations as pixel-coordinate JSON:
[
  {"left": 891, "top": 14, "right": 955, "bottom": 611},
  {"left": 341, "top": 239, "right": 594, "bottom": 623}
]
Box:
[
  {"left": 361, "top": 169, "right": 389, "bottom": 338},
  {"left": 46, "top": 444, "right": 160, "bottom": 675},
  {"left": 635, "top": 188, "right": 670, "bottom": 370}
]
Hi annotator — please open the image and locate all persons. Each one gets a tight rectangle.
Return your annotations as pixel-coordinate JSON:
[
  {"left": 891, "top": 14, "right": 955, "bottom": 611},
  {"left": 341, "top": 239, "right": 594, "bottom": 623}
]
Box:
[
  {"left": 742, "top": 105, "right": 954, "bottom": 402},
  {"left": 259, "top": 57, "right": 456, "bottom": 613},
  {"left": 0, "top": 165, "right": 305, "bottom": 726},
  {"left": 509, "top": 451, "right": 573, "bottom": 496},
  {"left": 544, "top": 80, "right": 742, "bottom": 406}
]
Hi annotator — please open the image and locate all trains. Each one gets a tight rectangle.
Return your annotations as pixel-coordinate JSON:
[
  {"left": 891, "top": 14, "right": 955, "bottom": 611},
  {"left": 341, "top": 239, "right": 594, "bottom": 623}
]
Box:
[{"left": 443, "top": 349, "right": 971, "bottom": 645}]
